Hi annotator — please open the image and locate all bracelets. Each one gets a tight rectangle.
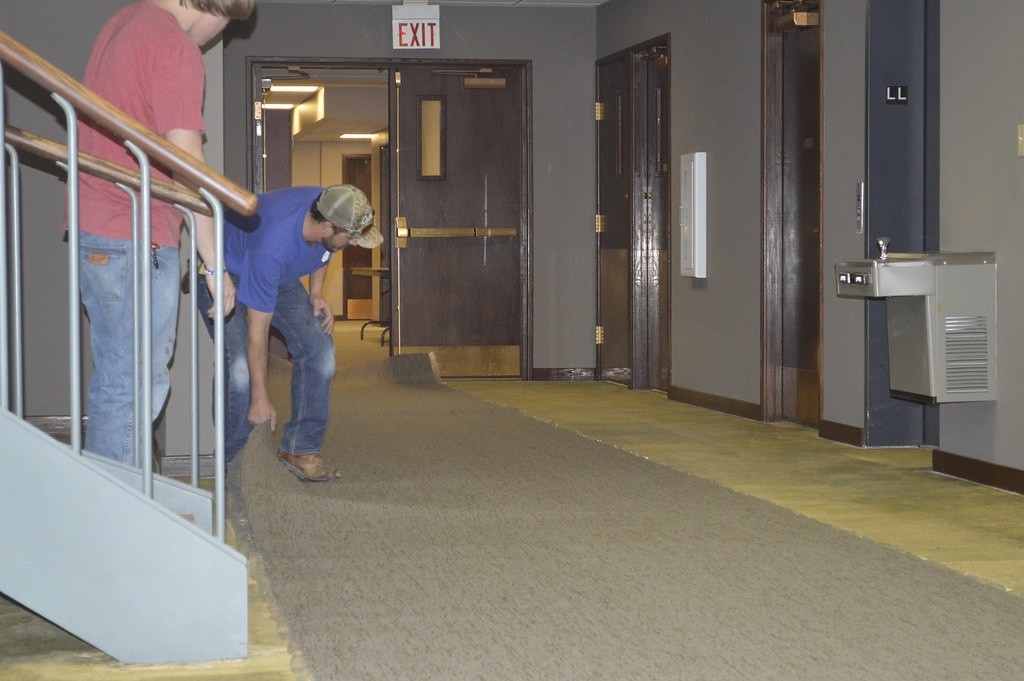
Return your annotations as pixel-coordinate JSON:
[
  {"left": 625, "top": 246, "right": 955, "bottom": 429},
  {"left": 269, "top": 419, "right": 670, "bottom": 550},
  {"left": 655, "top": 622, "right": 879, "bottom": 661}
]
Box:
[{"left": 204, "top": 265, "right": 229, "bottom": 275}]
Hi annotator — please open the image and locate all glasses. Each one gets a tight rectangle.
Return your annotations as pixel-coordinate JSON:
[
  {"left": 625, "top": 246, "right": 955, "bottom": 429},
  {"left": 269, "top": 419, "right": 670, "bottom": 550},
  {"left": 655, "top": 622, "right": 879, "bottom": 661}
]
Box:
[{"left": 333, "top": 209, "right": 375, "bottom": 234}]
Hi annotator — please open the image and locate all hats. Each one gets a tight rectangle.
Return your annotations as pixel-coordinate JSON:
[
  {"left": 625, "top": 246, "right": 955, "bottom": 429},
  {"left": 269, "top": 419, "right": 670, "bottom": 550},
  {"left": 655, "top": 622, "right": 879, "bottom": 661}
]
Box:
[{"left": 316, "top": 184, "right": 384, "bottom": 248}]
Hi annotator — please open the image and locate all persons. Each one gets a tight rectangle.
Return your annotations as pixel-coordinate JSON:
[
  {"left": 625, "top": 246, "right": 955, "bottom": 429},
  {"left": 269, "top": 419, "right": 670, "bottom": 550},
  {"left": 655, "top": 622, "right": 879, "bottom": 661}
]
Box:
[
  {"left": 197, "top": 185, "right": 384, "bottom": 482},
  {"left": 63, "top": 0, "right": 256, "bottom": 470}
]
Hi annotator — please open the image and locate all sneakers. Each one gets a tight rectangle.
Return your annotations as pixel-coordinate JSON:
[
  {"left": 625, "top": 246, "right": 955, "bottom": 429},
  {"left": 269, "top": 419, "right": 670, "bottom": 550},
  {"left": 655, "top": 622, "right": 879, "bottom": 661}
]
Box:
[{"left": 276, "top": 447, "right": 329, "bottom": 482}]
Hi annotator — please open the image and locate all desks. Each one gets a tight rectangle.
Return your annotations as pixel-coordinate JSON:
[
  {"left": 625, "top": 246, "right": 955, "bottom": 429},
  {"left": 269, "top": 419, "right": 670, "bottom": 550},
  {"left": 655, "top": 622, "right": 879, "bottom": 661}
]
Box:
[{"left": 351, "top": 267, "right": 391, "bottom": 347}]
